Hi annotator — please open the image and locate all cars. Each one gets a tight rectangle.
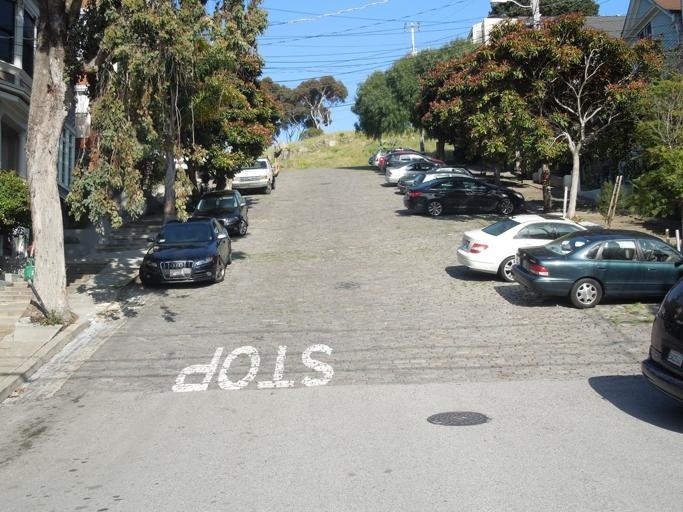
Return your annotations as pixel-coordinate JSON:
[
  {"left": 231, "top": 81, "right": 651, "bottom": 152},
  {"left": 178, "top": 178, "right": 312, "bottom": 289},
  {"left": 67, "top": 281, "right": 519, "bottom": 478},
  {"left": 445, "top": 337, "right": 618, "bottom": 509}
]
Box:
[
  {"left": 457, "top": 213, "right": 605, "bottom": 282},
  {"left": 512, "top": 228, "right": 683, "bottom": 308},
  {"left": 232, "top": 159, "right": 275, "bottom": 194},
  {"left": 187, "top": 190, "right": 248, "bottom": 236},
  {"left": 641, "top": 274, "right": 683, "bottom": 406},
  {"left": 369, "top": 146, "right": 524, "bottom": 215},
  {"left": 139, "top": 217, "right": 231, "bottom": 284}
]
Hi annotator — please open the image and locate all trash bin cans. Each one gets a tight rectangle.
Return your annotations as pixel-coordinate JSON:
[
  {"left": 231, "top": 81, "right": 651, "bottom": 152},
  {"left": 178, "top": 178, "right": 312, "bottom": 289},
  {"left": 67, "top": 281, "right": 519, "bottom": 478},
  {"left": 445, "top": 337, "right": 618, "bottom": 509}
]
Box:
[{"left": 25, "top": 260, "right": 36, "bottom": 282}]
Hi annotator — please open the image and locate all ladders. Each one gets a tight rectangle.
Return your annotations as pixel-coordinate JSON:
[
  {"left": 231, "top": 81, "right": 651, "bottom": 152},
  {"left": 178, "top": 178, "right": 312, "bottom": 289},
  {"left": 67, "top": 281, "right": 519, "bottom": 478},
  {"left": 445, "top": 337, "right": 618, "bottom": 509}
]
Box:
[{"left": 606, "top": 175, "right": 624, "bottom": 229}]
